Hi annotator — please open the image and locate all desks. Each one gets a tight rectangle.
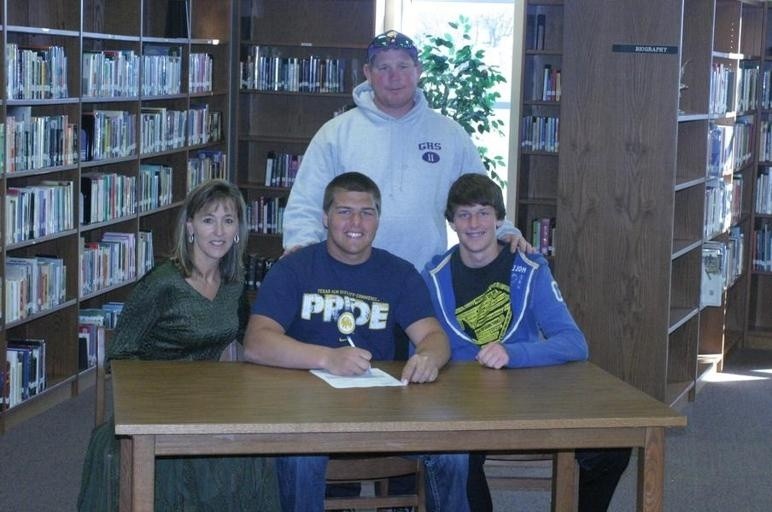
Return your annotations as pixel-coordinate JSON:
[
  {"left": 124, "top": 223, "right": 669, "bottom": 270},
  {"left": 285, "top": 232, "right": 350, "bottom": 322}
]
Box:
[{"left": 110, "top": 357, "right": 686, "bottom": 512}]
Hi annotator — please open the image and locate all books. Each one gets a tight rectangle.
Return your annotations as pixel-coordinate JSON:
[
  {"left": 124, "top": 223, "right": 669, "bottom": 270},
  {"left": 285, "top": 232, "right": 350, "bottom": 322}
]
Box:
[
  {"left": 239, "top": 45, "right": 345, "bottom": 93},
  {"left": 265, "top": 151, "right": 304, "bottom": 188},
  {"left": 521, "top": 13, "right": 563, "bottom": 260},
  {"left": 241, "top": 249, "right": 275, "bottom": 291},
  {"left": 2, "top": 44, "right": 78, "bottom": 409},
  {"left": 245, "top": 197, "right": 286, "bottom": 234},
  {"left": 81, "top": 230, "right": 154, "bottom": 297},
  {"left": 77, "top": 302, "right": 124, "bottom": 368},
  {"left": 694, "top": 57, "right": 771, "bottom": 316},
  {"left": 81, "top": 49, "right": 228, "bottom": 225}
]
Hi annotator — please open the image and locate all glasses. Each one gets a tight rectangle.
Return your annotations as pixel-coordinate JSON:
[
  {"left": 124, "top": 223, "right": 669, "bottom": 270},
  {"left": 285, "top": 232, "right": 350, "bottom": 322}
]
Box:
[{"left": 366, "top": 35, "right": 414, "bottom": 55}]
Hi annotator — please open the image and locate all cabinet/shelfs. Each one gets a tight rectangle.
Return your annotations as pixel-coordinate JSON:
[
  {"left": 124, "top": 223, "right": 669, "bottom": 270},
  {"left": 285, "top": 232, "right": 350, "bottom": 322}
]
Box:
[
  {"left": 236, "top": 3, "right": 374, "bottom": 292},
  {"left": 514, "top": 0, "right": 772, "bottom": 409},
  {"left": 5, "top": 2, "right": 235, "bottom": 421}
]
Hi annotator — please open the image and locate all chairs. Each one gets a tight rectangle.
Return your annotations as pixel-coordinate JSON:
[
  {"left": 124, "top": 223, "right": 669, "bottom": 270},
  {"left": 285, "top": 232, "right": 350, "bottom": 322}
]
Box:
[
  {"left": 239, "top": 289, "right": 425, "bottom": 511},
  {"left": 485, "top": 450, "right": 575, "bottom": 511}
]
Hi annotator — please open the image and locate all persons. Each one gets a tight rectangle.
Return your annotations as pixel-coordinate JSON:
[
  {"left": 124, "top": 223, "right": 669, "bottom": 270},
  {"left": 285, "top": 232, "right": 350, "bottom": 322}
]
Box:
[
  {"left": 405, "top": 172, "right": 635, "bottom": 511},
  {"left": 241, "top": 169, "right": 473, "bottom": 511},
  {"left": 281, "top": 29, "right": 540, "bottom": 360},
  {"left": 76, "top": 178, "right": 283, "bottom": 512}
]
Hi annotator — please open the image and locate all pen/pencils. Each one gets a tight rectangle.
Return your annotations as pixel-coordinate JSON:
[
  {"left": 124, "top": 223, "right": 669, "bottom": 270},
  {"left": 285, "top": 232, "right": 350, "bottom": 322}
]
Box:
[{"left": 347, "top": 336, "right": 372, "bottom": 375}]
